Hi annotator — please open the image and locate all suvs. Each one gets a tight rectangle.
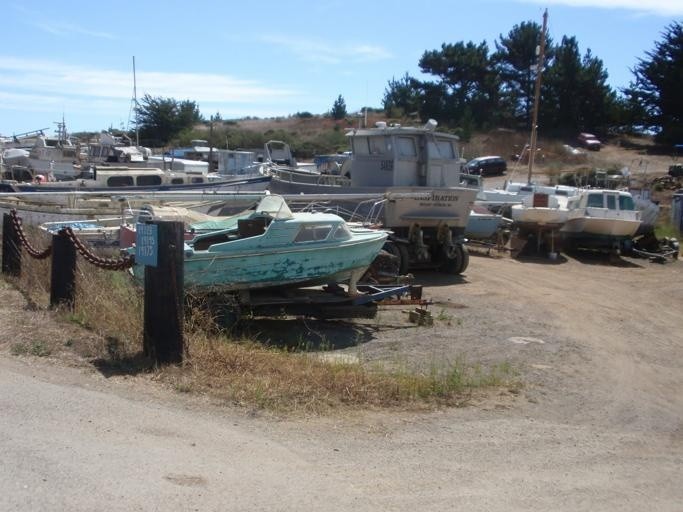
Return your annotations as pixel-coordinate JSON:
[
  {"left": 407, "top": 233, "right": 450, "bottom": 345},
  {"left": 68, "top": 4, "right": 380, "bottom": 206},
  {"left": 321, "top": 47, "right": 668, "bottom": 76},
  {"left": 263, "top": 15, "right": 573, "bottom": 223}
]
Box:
[
  {"left": 511, "top": 147, "right": 547, "bottom": 166},
  {"left": 577, "top": 132, "right": 603, "bottom": 152}
]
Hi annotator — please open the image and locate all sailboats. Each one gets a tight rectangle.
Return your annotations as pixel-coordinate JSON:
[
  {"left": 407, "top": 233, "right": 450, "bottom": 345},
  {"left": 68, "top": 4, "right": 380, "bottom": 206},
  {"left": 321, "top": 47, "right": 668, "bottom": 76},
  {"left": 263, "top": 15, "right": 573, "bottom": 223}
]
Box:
[{"left": 480, "top": 8, "right": 647, "bottom": 240}]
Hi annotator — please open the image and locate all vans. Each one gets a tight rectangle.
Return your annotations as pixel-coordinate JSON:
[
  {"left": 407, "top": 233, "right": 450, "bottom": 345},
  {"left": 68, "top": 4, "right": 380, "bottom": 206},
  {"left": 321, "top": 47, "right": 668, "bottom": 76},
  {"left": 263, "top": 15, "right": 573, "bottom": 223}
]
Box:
[{"left": 461, "top": 153, "right": 507, "bottom": 180}]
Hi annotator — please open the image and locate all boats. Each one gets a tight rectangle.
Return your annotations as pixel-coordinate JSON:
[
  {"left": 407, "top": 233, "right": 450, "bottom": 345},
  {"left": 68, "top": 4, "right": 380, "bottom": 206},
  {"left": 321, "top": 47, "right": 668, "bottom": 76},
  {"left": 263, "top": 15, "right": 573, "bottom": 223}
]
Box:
[{"left": 0, "top": 57, "right": 501, "bottom": 308}]
